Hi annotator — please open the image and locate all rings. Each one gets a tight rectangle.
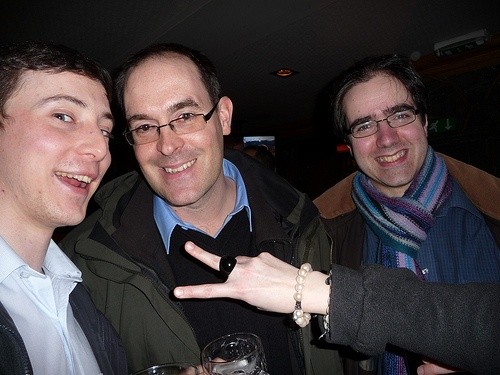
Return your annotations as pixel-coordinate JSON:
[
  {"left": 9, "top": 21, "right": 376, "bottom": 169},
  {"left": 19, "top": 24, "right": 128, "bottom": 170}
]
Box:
[{"left": 219, "top": 256, "right": 237, "bottom": 275}]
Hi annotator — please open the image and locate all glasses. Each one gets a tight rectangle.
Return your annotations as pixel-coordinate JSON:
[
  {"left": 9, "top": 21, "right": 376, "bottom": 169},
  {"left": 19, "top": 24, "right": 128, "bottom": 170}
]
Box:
[
  {"left": 346, "top": 108, "right": 422, "bottom": 139},
  {"left": 120, "top": 101, "right": 219, "bottom": 147}
]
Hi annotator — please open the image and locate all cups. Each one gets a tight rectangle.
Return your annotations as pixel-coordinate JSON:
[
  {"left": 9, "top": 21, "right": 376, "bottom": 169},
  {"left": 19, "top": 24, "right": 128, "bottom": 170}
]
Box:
[
  {"left": 201, "top": 332, "right": 268, "bottom": 375},
  {"left": 129, "top": 362, "right": 202, "bottom": 375}
]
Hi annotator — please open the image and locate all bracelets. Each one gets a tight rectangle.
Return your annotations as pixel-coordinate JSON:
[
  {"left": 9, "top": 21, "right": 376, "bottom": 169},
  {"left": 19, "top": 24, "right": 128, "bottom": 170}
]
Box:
[
  {"left": 293, "top": 263, "right": 313, "bottom": 328},
  {"left": 323, "top": 269, "right": 333, "bottom": 332}
]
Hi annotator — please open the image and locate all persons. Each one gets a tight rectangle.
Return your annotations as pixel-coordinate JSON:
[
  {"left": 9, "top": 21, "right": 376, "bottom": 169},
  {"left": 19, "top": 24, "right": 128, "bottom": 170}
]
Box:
[
  {"left": 0, "top": 41, "right": 132, "bottom": 375},
  {"left": 58, "top": 42, "right": 343, "bottom": 375},
  {"left": 313, "top": 57, "right": 500, "bottom": 375},
  {"left": 173, "top": 241, "right": 500, "bottom": 375}
]
